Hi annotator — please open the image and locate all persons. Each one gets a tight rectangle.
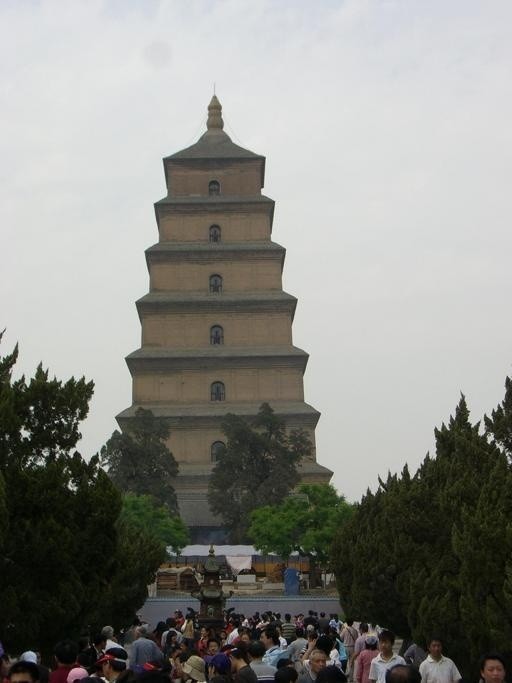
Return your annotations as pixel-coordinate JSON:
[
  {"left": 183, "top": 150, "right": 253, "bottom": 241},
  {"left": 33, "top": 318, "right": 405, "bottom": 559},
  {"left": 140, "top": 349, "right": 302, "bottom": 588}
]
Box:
[
  {"left": 1, "top": 605, "right": 462, "bottom": 683},
  {"left": 479, "top": 652, "right": 506, "bottom": 683}
]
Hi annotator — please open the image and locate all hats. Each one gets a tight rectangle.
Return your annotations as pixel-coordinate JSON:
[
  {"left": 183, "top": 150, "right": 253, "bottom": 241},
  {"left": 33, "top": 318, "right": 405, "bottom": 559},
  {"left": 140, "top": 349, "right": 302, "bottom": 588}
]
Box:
[
  {"left": 366, "top": 633, "right": 378, "bottom": 645},
  {"left": 94, "top": 654, "right": 127, "bottom": 666},
  {"left": 181, "top": 656, "right": 207, "bottom": 682},
  {"left": 19, "top": 651, "right": 37, "bottom": 664},
  {"left": 131, "top": 663, "right": 164, "bottom": 672},
  {"left": 67, "top": 668, "right": 88, "bottom": 683},
  {"left": 204, "top": 653, "right": 231, "bottom": 673}
]
